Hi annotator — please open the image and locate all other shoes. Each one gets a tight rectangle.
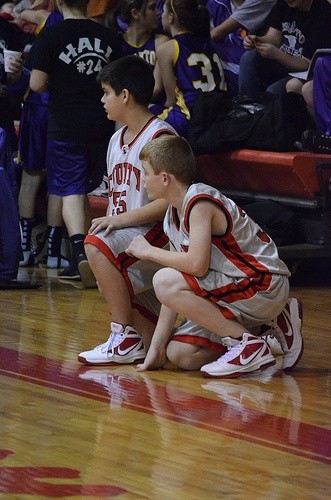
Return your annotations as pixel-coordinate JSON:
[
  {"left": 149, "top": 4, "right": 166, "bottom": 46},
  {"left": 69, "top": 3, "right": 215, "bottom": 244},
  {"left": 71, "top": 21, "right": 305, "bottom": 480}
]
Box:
[
  {"left": 19, "top": 251, "right": 38, "bottom": 266},
  {"left": 77, "top": 253, "right": 96, "bottom": 288},
  {"left": 58, "top": 261, "right": 80, "bottom": 279},
  {"left": 47, "top": 255, "right": 69, "bottom": 267}
]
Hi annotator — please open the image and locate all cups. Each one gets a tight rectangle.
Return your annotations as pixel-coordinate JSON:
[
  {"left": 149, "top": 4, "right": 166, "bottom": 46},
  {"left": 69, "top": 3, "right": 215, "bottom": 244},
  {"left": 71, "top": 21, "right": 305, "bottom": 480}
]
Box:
[{"left": 3, "top": 50, "right": 22, "bottom": 73}]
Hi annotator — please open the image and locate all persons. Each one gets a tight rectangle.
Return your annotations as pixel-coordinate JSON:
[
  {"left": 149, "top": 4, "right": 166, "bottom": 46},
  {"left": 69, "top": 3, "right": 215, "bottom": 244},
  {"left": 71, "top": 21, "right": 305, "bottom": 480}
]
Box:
[
  {"left": 0, "top": 0, "right": 42, "bottom": 37},
  {"left": 124, "top": 133, "right": 305, "bottom": 377},
  {"left": 90, "top": 0, "right": 229, "bottom": 196},
  {"left": 104, "top": 0, "right": 169, "bottom": 102},
  {"left": 16, "top": 1, "right": 73, "bottom": 269},
  {"left": 205, "top": 1, "right": 331, "bottom": 156},
  {"left": 20, "top": 290, "right": 303, "bottom": 499},
  {"left": 0, "top": 11, "right": 42, "bottom": 291},
  {"left": 28, "top": 0, "right": 128, "bottom": 287},
  {"left": 77, "top": 54, "right": 182, "bottom": 367}
]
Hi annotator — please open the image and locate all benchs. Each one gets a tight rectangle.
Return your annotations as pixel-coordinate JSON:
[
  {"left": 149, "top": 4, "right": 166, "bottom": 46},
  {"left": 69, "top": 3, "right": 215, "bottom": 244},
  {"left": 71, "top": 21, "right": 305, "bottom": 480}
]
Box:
[{"left": 86, "top": 149, "right": 331, "bottom": 268}]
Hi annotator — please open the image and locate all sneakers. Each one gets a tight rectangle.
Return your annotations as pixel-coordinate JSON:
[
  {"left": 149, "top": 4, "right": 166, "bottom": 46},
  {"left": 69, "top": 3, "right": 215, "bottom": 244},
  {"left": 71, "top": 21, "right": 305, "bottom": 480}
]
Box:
[
  {"left": 200, "top": 331, "right": 276, "bottom": 378},
  {"left": 78, "top": 321, "right": 146, "bottom": 365},
  {"left": 260, "top": 297, "right": 305, "bottom": 374}
]
